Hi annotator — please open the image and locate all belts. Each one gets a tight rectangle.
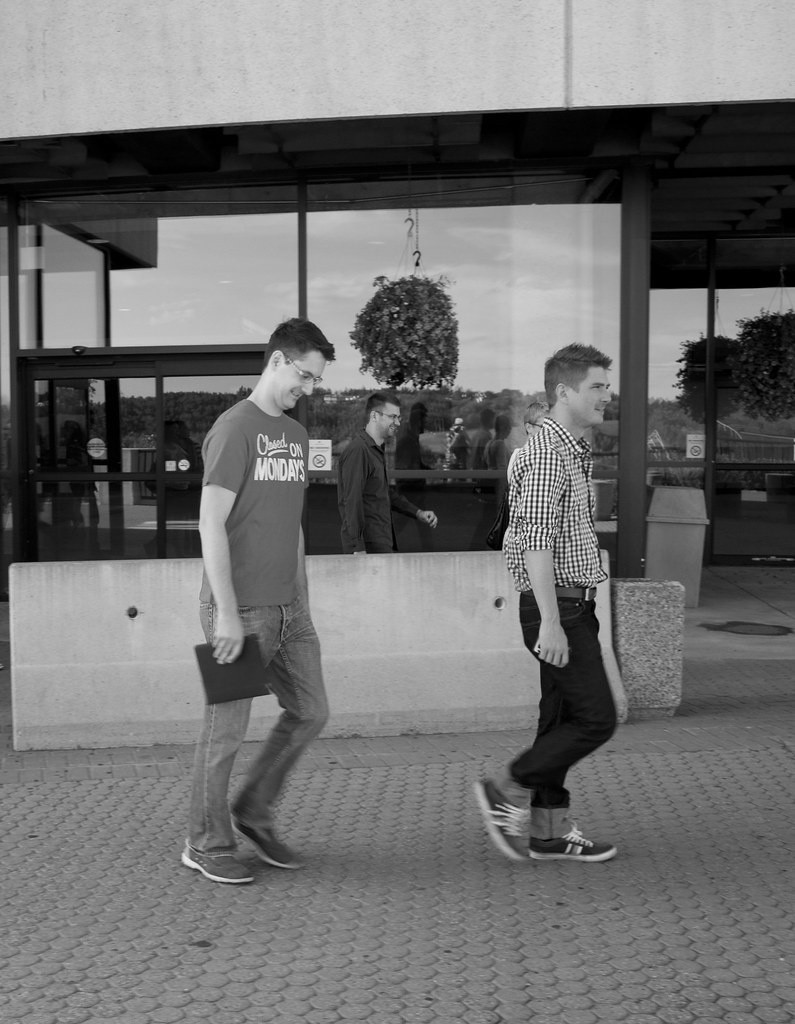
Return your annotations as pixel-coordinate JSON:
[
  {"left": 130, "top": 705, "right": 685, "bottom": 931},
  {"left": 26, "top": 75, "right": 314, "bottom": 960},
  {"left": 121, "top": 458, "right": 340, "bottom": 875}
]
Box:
[{"left": 522, "top": 587, "right": 597, "bottom": 602}]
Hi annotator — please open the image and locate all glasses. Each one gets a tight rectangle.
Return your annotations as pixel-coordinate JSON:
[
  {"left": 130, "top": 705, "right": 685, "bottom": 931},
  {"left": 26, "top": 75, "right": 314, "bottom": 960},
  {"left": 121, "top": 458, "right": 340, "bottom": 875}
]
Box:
[
  {"left": 375, "top": 410, "right": 402, "bottom": 422},
  {"left": 286, "top": 357, "right": 323, "bottom": 386}
]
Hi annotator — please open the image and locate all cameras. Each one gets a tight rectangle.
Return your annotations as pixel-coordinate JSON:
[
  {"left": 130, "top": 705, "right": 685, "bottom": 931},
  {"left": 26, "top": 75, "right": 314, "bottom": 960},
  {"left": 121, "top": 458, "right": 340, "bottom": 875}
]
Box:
[{"left": 454, "top": 426, "right": 461, "bottom": 433}]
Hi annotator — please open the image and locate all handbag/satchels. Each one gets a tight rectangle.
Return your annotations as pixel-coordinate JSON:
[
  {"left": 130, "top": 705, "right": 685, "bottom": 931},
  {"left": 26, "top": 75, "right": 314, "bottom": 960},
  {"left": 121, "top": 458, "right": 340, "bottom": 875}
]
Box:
[{"left": 485, "top": 489, "right": 511, "bottom": 550}]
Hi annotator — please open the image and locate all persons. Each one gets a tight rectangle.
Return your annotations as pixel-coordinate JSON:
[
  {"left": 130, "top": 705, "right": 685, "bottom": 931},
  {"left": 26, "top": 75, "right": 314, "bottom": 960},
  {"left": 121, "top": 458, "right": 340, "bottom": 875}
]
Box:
[
  {"left": 474, "top": 340, "right": 619, "bottom": 864},
  {"left": 394, "top": 401, "right": 518, "bottom": 501},
  {"left": 506, "top": 400, "right": 551, "bottom": 492},
  {"left": 337, "top": 392, "right": 438, "bottom": 556},
  {"left": 180, "top": 318, "right": 338, "bottom": 885}
]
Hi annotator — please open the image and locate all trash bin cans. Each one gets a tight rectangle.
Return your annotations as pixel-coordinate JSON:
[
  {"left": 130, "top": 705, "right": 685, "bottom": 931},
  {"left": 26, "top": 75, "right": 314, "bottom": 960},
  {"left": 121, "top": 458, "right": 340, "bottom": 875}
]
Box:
[{"left": 645, "top": 486, "right": 710, "bottom": 609}]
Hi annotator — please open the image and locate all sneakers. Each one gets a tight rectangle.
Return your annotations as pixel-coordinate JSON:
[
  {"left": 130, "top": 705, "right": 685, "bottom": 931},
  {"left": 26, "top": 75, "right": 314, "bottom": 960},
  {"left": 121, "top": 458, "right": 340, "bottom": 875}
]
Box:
[
  {"left": 231, "top": 818, "right": 305, "bottom": 869},
  {"left": 528, "top": 821, "right": 618, "bottom": 863},
  {"left": 473, "top": 779, "right": 530, "bottom": 863},
  {"left": 181, "top": 846, "right": 255, "bottom": 883}
]
